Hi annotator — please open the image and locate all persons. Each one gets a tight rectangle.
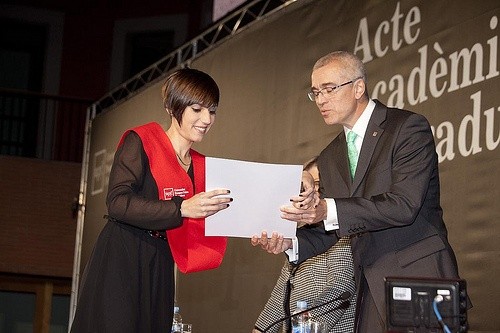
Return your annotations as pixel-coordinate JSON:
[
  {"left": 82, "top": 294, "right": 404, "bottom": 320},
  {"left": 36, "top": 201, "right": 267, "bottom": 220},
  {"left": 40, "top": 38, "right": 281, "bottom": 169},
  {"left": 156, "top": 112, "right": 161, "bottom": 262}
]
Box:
[
  {"left": 70, "top": 68, "right": 233, "bottom": 333},
  {"left": 254, "top": 160, "right": 358, "bottom": 333},
  {"left": 250, "top": 51, "right": 474, "bottom": 333}
]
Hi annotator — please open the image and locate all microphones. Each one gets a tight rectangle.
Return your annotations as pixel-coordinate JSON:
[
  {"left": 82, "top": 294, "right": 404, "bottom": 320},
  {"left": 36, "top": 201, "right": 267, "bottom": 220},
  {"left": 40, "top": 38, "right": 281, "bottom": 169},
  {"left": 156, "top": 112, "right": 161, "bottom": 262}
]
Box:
[{"left": 263, "top": 291, "right": 351, "bottom": 333}]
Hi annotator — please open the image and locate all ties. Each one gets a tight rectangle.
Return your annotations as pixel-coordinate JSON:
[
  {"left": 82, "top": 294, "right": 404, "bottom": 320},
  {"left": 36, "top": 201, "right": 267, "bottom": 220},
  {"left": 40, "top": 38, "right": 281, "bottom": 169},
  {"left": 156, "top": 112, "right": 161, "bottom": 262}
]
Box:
[{"left": 346, "top": 132, "right": 359, "bottom": 181}]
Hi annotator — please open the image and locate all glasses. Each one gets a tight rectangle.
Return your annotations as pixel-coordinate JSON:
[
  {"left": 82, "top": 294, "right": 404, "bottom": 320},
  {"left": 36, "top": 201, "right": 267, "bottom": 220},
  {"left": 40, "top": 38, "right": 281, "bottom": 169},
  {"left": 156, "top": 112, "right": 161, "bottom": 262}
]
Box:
[{"left": 307, "top": 77, "right": 363, "bottom": 101}]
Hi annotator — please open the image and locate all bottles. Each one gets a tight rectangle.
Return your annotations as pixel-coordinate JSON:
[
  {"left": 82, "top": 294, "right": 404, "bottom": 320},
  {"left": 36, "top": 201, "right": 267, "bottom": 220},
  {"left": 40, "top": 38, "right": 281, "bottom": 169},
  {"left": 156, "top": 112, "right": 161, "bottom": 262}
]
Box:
[
  {"left": 292, "top": 301, "right": 311, "bottom": 333},
  {"left": 171, "top": 307, "right": 182, "bottom": 333}
]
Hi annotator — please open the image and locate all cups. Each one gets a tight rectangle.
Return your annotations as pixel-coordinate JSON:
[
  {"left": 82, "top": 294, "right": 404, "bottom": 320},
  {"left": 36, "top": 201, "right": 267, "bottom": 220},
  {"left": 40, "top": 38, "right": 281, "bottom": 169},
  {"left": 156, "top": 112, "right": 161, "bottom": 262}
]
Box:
[
  {"left": 181, "top": 324, "right": 192, "bottom": 333},
  {"left": 311, "top": 322, "right": 327, "bottom": 333}
]
